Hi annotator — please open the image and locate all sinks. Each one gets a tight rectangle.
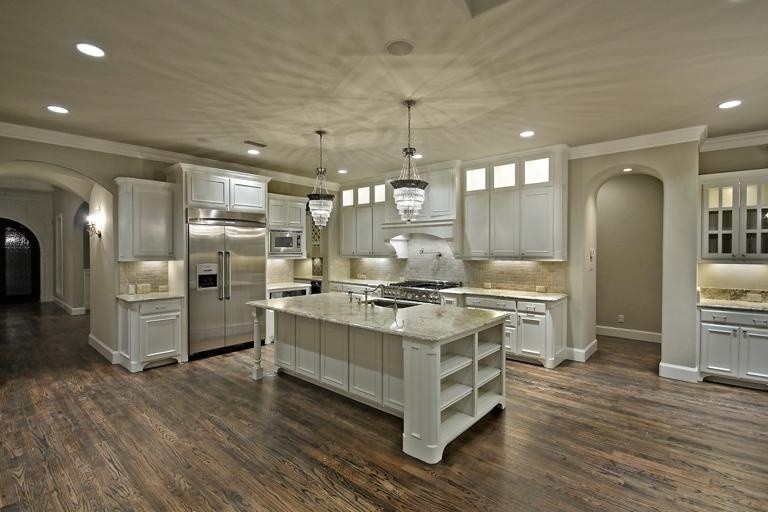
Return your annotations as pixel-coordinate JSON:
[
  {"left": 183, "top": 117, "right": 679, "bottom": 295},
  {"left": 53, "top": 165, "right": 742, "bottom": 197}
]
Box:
[
  {"left": 388, "top": 304, "right": 416, "bottom": 308},
  {"left": 365, "top": 299, "right": 393, "bottom": 307}
]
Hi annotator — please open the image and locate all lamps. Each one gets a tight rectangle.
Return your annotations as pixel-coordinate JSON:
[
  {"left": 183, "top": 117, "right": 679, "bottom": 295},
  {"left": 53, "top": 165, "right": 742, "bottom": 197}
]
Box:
[
  {"left": 390, "top": 99, "right": 429, "bottom": 225},
  {"left": 306, "top": 127, "right": 336, "bottom": 230}
]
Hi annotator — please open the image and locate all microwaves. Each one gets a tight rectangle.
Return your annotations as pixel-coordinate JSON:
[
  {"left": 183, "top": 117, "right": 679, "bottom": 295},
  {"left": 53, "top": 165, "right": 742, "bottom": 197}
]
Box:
[{"left": 270, "top": 230, "right": 303, "bottom": 254}]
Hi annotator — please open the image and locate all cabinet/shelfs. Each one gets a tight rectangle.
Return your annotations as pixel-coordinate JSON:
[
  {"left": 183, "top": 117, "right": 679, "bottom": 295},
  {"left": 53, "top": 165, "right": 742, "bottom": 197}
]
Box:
[
  {"left": 266, "top": 192, "right": 309, "bottom": 260},
  {"left": 699, "top": 307, "right": 768, "bottom": 390},
  {"left": 697, "top": 176, "right": 767, "bottom": 260},
  {"left": 337, "top": 202, "right": 388, "bottom": 258},
  {"left": 384, "top": 158, "right": 458, "bottom": 231},
  {"left": 171, "top": 162, "right": 273, "bottom": 215},
  {"left": 401, "top": 316, "right": 508, "bottom": 465},
  {"left": 465, "top": 184, "right": 559, "bottom": 260},
  {"left": 463, "top": 297, "right": 567, "bottom": 368},
  {"left": 117, "top": 300, "right": 189, "bottom": 374},
  {"left": 112, "top": 176, "right": 175, "bottom": 262}
]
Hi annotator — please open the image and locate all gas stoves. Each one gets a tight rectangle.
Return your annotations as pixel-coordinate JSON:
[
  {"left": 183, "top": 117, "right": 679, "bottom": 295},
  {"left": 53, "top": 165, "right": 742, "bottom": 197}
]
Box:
[{"left": 380, "top": 279, "right": 460, "bottom": 304}]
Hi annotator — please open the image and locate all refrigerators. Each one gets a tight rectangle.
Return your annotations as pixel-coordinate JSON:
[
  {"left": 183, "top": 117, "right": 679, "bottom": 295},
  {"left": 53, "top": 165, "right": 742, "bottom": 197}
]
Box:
[{"left": 185, "top": 205, "right": 266, "bottom": 357}]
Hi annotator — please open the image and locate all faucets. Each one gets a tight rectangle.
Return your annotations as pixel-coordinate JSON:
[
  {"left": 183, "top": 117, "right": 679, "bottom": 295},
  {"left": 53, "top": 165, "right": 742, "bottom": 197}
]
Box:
[{"left": 364, "top": 284, "right": 386, "bottom": 301}]
